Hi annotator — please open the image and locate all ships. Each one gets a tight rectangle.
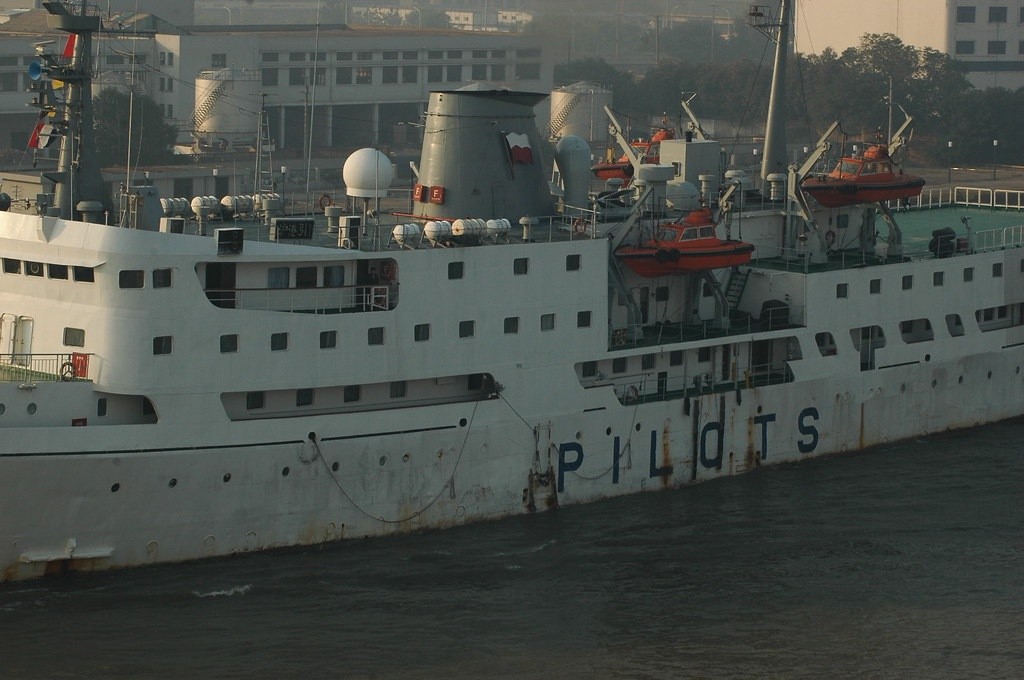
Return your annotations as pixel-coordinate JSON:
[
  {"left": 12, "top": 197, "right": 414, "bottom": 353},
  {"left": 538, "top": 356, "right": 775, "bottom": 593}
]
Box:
[{"left": 0, "top": 1, "right": 1024, "bottom": 576}]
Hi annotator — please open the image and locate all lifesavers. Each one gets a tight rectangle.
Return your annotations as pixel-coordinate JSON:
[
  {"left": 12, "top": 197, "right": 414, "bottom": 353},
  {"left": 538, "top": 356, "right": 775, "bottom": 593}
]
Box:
[
  {"left": 823, "top": 230, "right": 838, "bottom": 248},
  {"left": 319, "top": 193, "right": 332, "bottom": 211},
  {"left": 57, "top": 360, "right": 79, "bottom": 385},
  {"left": 573, "top": 218, "right": 587, "bottom": 237}
]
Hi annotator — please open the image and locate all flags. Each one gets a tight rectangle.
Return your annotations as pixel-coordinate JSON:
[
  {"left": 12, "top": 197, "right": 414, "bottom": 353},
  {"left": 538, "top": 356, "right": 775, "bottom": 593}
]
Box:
[{"left": 26, "top": 123, "right": 59, "bottom": 149}]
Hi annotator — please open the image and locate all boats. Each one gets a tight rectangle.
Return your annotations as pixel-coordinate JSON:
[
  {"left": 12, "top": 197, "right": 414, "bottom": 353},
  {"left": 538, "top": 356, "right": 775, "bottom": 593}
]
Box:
[
  {"left": 613, "top": 191, "right": 757, "bottom": 278},
  {"left": 588, "top": 124, "right": 675, "bottom": 183},
  {"left": 798, "top": 140, "right": 928, "bottom": 210}
]
